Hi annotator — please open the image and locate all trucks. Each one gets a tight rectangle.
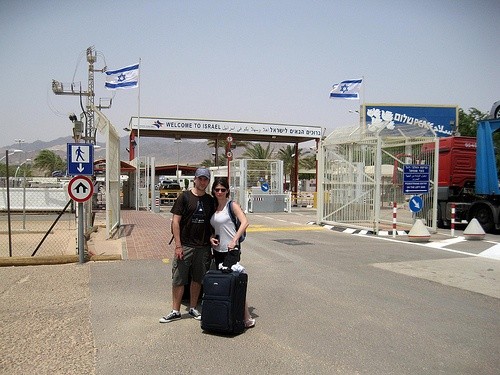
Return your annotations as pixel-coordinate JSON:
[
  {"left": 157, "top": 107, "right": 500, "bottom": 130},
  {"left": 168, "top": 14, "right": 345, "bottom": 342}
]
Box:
[{"left": 418, "top": 118, "right": 500, "bottom": 236}]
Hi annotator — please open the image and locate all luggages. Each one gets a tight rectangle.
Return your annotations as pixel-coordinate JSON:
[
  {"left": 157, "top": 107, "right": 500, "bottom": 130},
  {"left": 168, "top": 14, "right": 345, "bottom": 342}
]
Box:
[{"left": 200, "top": 245, "right": 248, "bottom": 338}]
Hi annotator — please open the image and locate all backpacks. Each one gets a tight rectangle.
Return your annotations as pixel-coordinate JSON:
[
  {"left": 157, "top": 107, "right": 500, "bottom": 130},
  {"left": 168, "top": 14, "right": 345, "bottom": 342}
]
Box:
[
  {"left": 227, "top": 200, "right": 246, "bottom": 243},
  {"left": 168, "top": 187, "right": 190, "bottom": 245}
]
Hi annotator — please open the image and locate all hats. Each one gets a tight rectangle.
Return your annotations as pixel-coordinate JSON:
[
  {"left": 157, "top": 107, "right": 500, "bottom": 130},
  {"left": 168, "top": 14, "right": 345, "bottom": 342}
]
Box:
[{"left": 194, "top": 167, "right": 210, "bottom": 179}]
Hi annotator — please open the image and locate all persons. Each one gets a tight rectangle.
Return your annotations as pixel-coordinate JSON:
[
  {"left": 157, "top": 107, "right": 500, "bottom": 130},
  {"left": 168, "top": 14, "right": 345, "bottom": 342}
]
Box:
[
  {"left": 210, "top": 177, "right": 256, "bottom": 328},
  {"left": 160, "top": 167, "right": 214, "bottom": 323}
]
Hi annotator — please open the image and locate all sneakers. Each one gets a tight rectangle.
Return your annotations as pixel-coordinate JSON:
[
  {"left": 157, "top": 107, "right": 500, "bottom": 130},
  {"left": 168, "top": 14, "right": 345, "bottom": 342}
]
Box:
[
  {"left": 188, "top": 309, "right": 201, "bottom": 320},
  {"left": 246, "top": 315, "right": 255, "bottom": 328},
  {"left": 159, "top": 311, "right": 181, "bottom": 323}
]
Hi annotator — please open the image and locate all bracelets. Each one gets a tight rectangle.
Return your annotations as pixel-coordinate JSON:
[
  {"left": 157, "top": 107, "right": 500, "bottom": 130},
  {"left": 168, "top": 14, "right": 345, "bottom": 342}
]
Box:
[{"left": 175, "top": 245, "right": 182, "bottom": 248}]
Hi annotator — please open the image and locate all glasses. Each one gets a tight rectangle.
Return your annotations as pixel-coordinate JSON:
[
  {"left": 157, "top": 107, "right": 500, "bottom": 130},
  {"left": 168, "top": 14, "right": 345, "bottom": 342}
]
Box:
[{"left": 214, "top": 189, "right": 227, "bottom": 192}]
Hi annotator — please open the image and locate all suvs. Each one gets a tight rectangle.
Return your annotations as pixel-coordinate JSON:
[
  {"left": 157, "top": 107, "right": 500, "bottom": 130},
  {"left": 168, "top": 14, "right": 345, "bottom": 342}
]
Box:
[{"left": 149, "top": 180, "right": 184, "bottom": 204}]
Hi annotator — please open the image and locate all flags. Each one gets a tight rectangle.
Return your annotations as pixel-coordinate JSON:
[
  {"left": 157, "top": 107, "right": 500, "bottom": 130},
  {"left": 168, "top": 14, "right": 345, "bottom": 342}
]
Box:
[
  {"left": 329, "top": 79, "right": 362, "bottom": 99},
  {"left": 104, "top": 63, "right": 138, "bottom": 90}
]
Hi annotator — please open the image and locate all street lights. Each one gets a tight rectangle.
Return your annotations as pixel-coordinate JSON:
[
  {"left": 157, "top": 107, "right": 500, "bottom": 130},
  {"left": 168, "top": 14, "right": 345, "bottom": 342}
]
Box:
[{"left": 0, "top": 138, "right": 31, "bottom": 177}]
